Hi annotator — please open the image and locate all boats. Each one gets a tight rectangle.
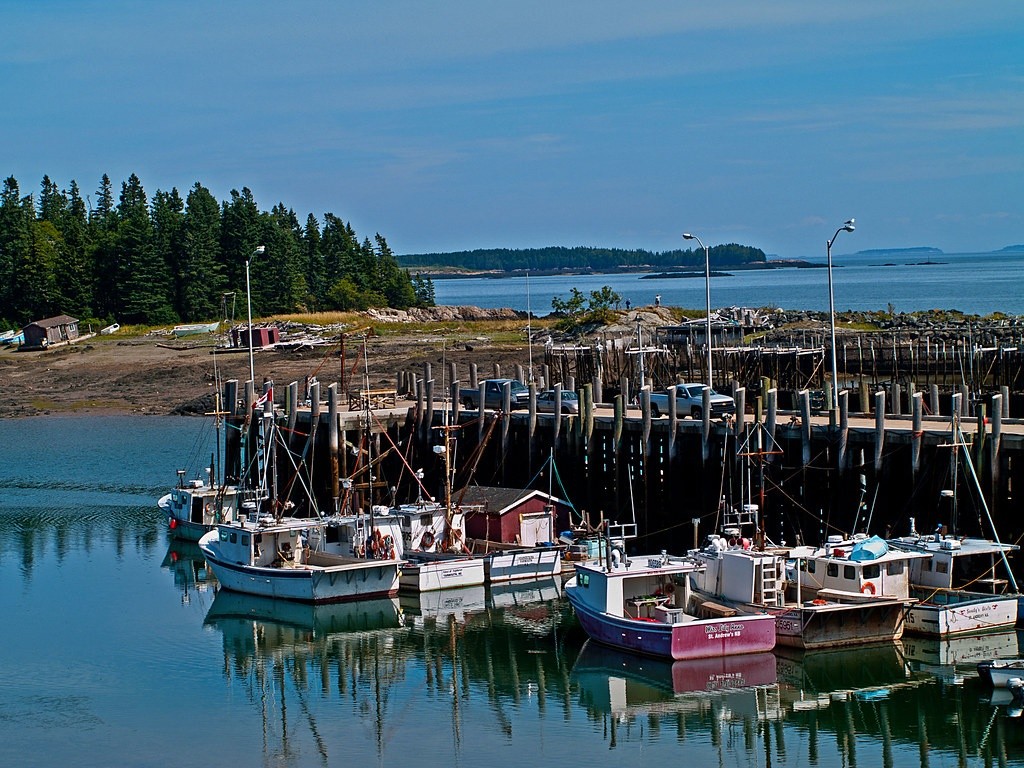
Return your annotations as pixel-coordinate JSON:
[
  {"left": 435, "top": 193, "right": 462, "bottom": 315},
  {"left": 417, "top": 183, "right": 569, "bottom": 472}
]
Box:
[
  {"left": 343, "top": 388, "right": 485, "bottom": 593},
  {"left": 685, "top": 422, "right": 920, "bottom": 653},
  {"left": 781, "top": 408, "right": 1020, "bottom": 639},
  {"left": 563, "top": 519, "right": 775, "bottom": 662},
  {"left": 157, "top": 370, "right": 263, "bottom": 542},
  {"left": 427, "top": 388, "right": 570, "bottom": 587},
  {"left": 198, "top": 377, "right": 408, "bottom": 606}
]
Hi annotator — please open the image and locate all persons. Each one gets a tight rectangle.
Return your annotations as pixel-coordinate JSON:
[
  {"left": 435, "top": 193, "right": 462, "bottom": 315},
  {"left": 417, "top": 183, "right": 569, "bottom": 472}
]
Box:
[
  {"left": 302, "top": 544, "right": 310, "bottom": 565},
  {"left": 625, "top": 298, "right": 631, "bottom": 310},
  {"left": 655, "top": 294, "right": 662, "bottom": 308}
]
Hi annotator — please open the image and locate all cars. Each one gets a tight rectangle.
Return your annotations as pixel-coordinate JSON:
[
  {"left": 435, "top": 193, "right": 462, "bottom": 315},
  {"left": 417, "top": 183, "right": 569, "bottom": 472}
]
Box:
[{"left": 527, "top": 389, "right": 598, "bottom": 414}]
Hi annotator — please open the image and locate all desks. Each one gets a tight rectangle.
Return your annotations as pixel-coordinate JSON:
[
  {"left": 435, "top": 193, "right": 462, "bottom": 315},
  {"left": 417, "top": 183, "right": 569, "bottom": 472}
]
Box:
[{"left": 626, "top": 594, "right": 671, "bottom": 618}]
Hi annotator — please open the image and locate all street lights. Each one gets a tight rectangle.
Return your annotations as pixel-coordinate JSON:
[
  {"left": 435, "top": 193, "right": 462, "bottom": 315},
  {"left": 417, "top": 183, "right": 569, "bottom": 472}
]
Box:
[
  {"left": 827, "top": 217, "right": 857, "bottom": 407},
  {"left": 682, "top": 232, "right": 713, "bottom": 388},
  {"left": 245, "top": 246, "right": 267, "bottom": 395}
]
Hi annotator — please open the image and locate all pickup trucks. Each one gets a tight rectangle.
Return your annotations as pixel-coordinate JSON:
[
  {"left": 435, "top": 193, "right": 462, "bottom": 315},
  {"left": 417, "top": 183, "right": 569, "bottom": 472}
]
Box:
[
  {"left": 459, "top": 377, "right": 529, "bottom": 412},
  {"left": 638, "top": 382, "right": 737, "bottom": 420}
]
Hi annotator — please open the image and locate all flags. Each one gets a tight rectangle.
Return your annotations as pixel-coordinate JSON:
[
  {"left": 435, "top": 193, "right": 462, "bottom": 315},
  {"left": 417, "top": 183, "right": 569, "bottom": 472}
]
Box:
[{"left": 251, "top": 387, "right": 272, "bottom": 410}]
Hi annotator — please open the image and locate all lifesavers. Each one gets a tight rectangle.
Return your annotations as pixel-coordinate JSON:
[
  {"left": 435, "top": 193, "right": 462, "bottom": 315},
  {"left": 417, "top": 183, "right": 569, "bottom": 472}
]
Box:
[
  {"left": 421, "top": 531, "right": 435, "bottom": 549},
  {"left": 205, "top": 501, "right": 217, "bottom": 516},
  {"left": 860, "top": 582, "right": 876, "bottom": 595}
]
